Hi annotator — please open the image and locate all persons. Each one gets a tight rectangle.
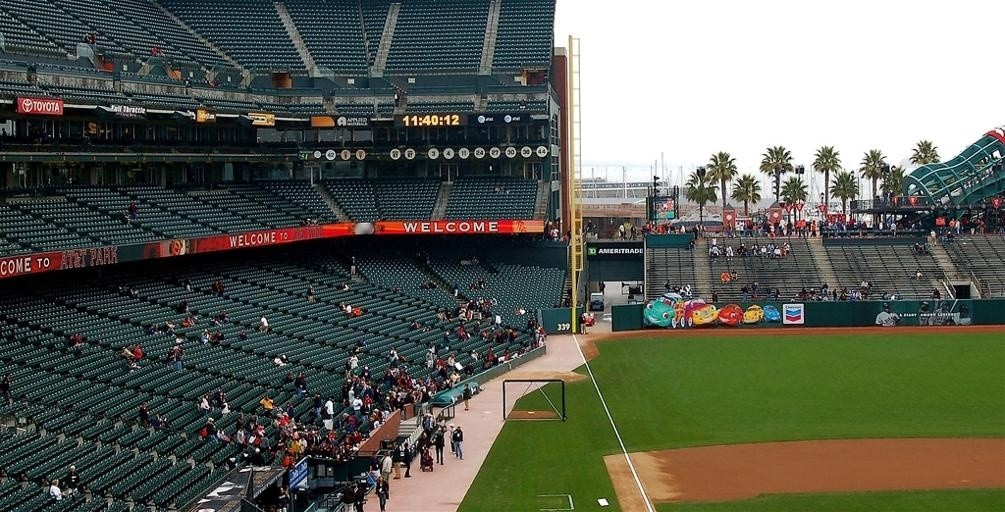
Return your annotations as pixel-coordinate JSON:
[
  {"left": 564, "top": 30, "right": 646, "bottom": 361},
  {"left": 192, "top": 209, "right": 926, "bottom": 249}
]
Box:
[
  {"left": 950, "top": 302, "right": 971, "bottom": 325},
  {"left": 740, "top": 278, "right": 875, "bottom": 304},
  {"left": 89, "top": 30, "right": 100, "bottom": 47},
  {"left": 918, "top": 302, "right": 931, "bottom": 325},
  {"left": 349, "top": 256, "right": 357, "bottom": 274},
  {"left": 719, "top": 210, "right": 1005, "bottom": 247},
  {"left": 915, "top": 269, "right": 923, "bottom": 280},
  {"left": 686, "top": 239, "right": 697, "bottom": 253},
  {"left": 598, "top": 281, "right": 606, "bottom": 293},
  {"left": 873, "top": 303, "right": 900, "bottom": 327},
  {"left": 881, "top": 290, "right": 888, "bottom": 299},
  {"left": 547, "top": 218, "right": 708, "bottom": 240},
  {"left": 83, "top": 32, "right": 91, "bottom": 47},
  {"left": 929, "top": 301, "right": 952, "bottom": 325},
  {"left": 665, "top": 280, "right": 671, "bottom": 294},
  {"left": 923, "top": 242, "right": 930, "bottom": 254},
  {"left": 49, "top": 479, "right": 63, "bottom": 502},
  {"left": 151, "top": 45, "right": 166, "bottom": 59},
  {"left": 553, "top": 302, "right": 561, "bottom": 308},
  {"left": 891, "top": 293, "right": 895, "bottom": 299},
  {"left": 721, "top": 268, "right": 739, "bottom": 286},
  {"left": 298, "top": 216, "right": 320, "bottom": 228},
  {"left": 712, "top": 291, "right": 718, "bottom": 302},
  {"left": 709, "top": 237, "right": 790, "bottom": 261},
  {"left": 673, "top": 282, "right": 693, "bottom": 299},
  {"left": 67, "top": 262, "right": 543, "bottom": 512},
  {"left": 128, "top": 202, "right": 137, "bottom": 219},
  {"left": 567, "top": 288, "right": 572, "bottom": 297},
  {"left": 459, "top": 253, "right": 481, "bottom": 271},
  {"left": 913, "top": 241, "right": 919, "bottom": 252},
  {"left": 579, "top": 314, "right": 587, "bottom": 335},
  {"left": 931, "top": 288, "right": 940, "bottom": 299},
  {"left": 565, "top": 296, "right": 570, "bottom": 307},
  {"left": 2, "top": 373, "right": 14, "bottom": 406}
]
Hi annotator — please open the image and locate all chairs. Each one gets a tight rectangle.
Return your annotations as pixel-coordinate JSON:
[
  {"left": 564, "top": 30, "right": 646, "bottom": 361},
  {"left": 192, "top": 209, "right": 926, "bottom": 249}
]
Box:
[
  {"left": 0, "top": 0, "right": 570, "bottom": 510},
  {"left": 648, "top": 241, "right": 996, "bottom": 312}
]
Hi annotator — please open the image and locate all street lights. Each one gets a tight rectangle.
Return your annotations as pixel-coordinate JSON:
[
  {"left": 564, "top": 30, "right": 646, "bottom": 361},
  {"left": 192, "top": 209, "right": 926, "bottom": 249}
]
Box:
[{"left": 654, "top": 177, "right": 660, "bottom": 233}]
